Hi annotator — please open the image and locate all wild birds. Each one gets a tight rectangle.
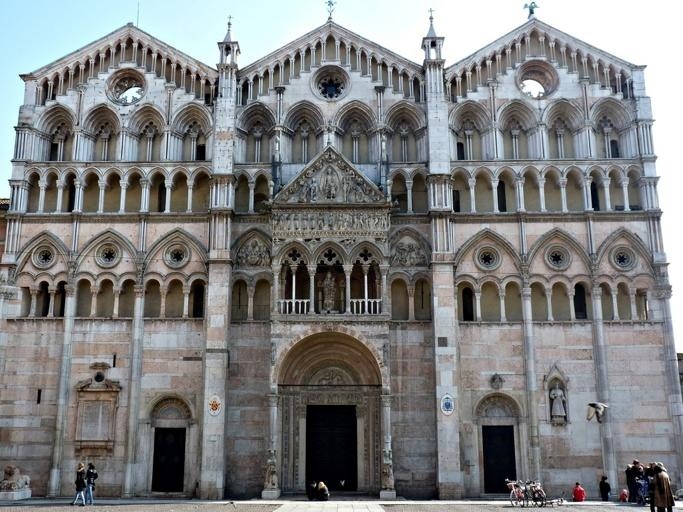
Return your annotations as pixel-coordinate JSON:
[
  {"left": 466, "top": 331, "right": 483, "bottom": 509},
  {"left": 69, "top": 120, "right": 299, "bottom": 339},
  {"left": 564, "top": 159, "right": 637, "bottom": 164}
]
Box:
[
  {"left": 584, "top": 400, "right": 608, "bottom": 423},
  {"left": 224, "top": 500, "right": 236, "bottom": 509}
]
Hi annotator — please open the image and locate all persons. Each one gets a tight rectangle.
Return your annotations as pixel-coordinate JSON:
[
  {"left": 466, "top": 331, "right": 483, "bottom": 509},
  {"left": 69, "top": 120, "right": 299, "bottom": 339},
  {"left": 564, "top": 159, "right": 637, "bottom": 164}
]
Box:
[
  {"left": 550, "top": 382, "right": 566, "bottom": 418},
  {"left": 70, "top": 463, "right": 87, "bottom": 506},
  {"left": 322, "top": 271, "right": 338, "bottom": 311},
  {"left": 619, "top": 458, "right": 675, "bottom": 512},
  {"left": 600, "top": 475, "right": 611, "bottom": 501},
  {"left": 572, "top": 482, "right": 586, "bottom": 501},
  {"left": 84, "top": 462, "right": 98, "bottom": 505},
  {"left": 318, "top": 482, "right": 329, "bottom": 501},
  {"left": 306, "top": 481, "right": 317, "bottom": 500}
]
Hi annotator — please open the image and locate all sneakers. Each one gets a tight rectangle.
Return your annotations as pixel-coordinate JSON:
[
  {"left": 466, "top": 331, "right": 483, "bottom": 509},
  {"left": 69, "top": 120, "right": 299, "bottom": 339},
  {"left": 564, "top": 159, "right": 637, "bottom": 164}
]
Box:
[{"left": 69, "top": 502, "right": 93, "bottom": 506}]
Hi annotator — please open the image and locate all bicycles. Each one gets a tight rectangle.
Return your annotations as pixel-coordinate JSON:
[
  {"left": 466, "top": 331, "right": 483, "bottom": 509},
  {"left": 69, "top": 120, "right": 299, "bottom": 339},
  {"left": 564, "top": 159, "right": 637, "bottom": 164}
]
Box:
[{"left": 503, "top": 476, "right": 546, "bottom": 509}]
